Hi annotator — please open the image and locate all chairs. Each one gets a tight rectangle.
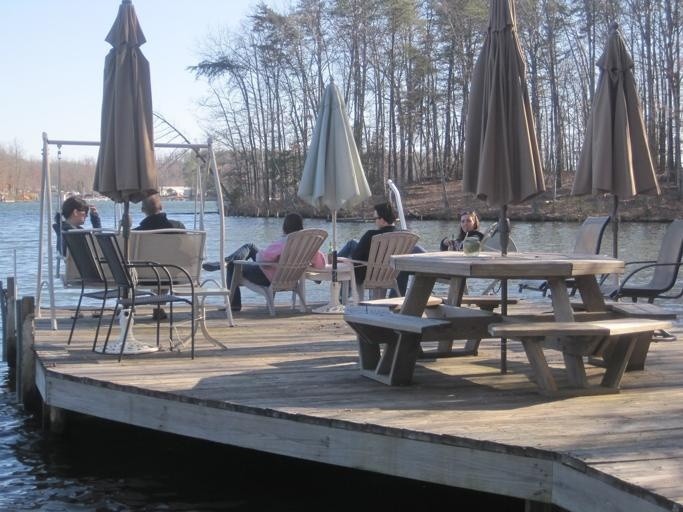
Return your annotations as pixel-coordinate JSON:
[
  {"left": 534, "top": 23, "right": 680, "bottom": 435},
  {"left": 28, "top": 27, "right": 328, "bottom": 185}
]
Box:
[
  {"left": 600, "top": 218, "right": 683, "bottom": 303},
  {"left": 519, "top": 216, "right": 611, "bottom": 298},
  {"left": 329, "top": 231, "right": 420, "bottom": 298},
  {"left": 436, "top": 217, "right": 518, "bottom": 307},
  {"left": 228, "top": 228, "right": 329, "bottom": 315},
  {"left": 62, "top": 230, "right": 195, "bottom": 362}
]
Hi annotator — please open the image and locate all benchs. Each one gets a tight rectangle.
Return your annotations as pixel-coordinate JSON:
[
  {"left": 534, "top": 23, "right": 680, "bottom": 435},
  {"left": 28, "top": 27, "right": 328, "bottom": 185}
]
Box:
[{"left": 59, "top": 228, "right": 206, "bottom": 290}]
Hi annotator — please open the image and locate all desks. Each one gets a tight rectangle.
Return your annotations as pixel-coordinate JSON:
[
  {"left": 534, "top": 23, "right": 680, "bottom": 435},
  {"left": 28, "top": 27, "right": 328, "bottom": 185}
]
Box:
[{"left": 390, "top": 250, "right": 626, "bottom": 387}]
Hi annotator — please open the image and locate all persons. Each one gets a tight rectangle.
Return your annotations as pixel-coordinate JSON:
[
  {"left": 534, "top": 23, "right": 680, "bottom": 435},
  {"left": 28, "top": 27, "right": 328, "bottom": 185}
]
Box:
[
  {"left": 389, "top": 211, "right": 485, "bottom": 299},
  {"left": 130, "top": 194, "right": 187, "bottom": 321},
  {"left": 52, "top": 198, "right": 102, "bottom": 258},
  {"left": 202, "top": 213, "right": 325, "bottom": 311},
  {"left": 313, "top": 202, "right": 409, "bottom": 304}
]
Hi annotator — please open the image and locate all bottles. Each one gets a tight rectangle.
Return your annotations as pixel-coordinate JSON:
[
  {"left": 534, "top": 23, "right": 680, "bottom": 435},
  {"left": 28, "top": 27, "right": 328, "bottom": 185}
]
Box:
[
  {"left": 448, "top": 235, "right": 455, "bottom": 251},
  {"left": 463, "top": 237, "right": 480, "bottom": 257},
  {"left": 327, "top": 241, "right": 333, "bottom": 265}
]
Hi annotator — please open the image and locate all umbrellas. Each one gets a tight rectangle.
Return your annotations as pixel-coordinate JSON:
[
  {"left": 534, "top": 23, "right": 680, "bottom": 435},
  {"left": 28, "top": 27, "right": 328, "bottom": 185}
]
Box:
[
  {"left": 461, "top": 0, "right": 546, "bottom": 375},
  {"left": 296, "top": 75, "right": 372, "bottom": 306},
  {"left": 92, "top": 0, "right": 159, "bottom": 338},
  {"left": 569, "top": 22, "right": 662, "bottom": 302}
]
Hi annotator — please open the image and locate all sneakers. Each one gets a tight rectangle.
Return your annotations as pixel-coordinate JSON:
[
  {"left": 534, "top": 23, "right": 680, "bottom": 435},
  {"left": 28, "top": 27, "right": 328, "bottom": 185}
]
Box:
[
  {"left": 153, "top": 309, "right": 167, "bottom": 319},
  {"left": 218, "top": 304, "right": 241, "bottom": 311},
  {"left": 202, "top": 260, "right": 227, "bottom": 271}
]
